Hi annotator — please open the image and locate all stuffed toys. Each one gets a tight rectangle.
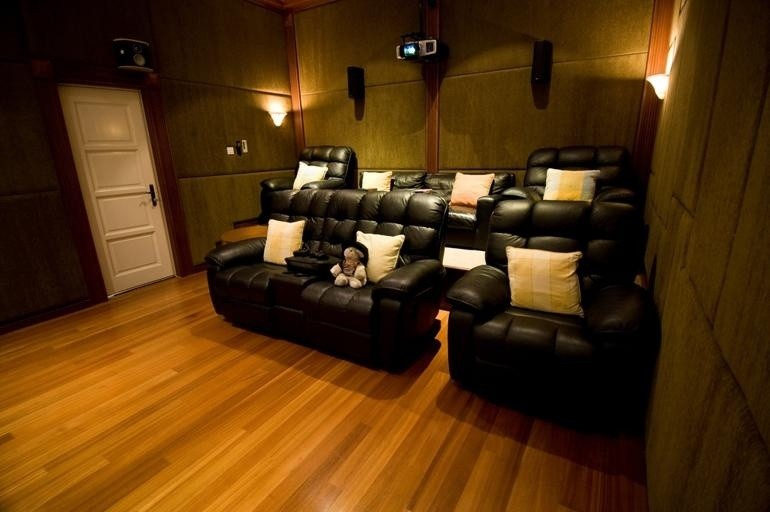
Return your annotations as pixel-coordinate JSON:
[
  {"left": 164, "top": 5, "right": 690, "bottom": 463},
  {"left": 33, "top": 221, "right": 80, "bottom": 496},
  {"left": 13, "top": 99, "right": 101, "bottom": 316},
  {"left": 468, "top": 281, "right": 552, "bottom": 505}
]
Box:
[{"left": 330, "top": 242, "right": 369, "bottom": 289}]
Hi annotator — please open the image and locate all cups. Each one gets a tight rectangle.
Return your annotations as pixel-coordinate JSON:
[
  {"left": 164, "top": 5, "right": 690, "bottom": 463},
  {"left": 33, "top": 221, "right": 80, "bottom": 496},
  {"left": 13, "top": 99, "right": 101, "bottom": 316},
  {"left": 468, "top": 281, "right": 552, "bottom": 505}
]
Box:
[{"left": 368, "top": 188, "right": 378, "bottom": 193}]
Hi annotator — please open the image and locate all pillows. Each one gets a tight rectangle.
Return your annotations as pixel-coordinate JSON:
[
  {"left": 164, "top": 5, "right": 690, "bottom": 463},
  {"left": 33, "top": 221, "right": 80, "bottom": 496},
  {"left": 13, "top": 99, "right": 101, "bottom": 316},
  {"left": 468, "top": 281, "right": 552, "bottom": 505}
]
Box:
[
  {"left": 352, "top": 224, "right": 406, "bottom": 288},
  {"left": 541, "top": 163, "right": 601, "bottom": 206},
  {"left": 261, "top": 213, "right": 307, "bottom": 267},
  {"left": 447, "top": 172, "right": 497, "bottom": 208},
  {"left": 359, "top": 171, "right": 394, "bottom": 194},
  {"left": 501, "top": 238, "right": 592, "bottom": 320},
  {"left": 292, "top": 160, "right": 329, "bottom": 190}
]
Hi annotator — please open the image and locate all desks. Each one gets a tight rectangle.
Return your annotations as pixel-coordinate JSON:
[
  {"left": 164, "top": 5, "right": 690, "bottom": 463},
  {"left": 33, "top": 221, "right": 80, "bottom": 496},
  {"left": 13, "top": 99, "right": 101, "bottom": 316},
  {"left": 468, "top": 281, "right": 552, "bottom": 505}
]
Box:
[{"left": 441, "top": 245, "right": 485, "bottom": 280}]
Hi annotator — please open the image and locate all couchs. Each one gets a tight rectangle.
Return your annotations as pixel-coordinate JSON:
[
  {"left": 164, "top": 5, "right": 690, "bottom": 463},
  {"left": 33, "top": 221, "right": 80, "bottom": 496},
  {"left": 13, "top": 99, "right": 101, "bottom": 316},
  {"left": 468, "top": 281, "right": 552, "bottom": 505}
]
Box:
[
  {"left": 475, "top": 145, "right": 634, "bottom": 236},
  {"left": 356, "top": 170, "right": 519, "bottom": 248},
  {"left": 204, "top": 180, "right": 454, "bottom": 376}
]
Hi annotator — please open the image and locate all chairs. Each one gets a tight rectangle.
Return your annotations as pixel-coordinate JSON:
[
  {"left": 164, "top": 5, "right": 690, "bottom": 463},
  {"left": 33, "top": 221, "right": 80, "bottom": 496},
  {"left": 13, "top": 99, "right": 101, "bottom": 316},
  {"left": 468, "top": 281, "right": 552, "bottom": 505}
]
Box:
[
  {"left": 258, "top": 146, "right": 358, "bottom": 222},
  {"left": 441, "top": 193, "right": 662, "bottom": 432}
]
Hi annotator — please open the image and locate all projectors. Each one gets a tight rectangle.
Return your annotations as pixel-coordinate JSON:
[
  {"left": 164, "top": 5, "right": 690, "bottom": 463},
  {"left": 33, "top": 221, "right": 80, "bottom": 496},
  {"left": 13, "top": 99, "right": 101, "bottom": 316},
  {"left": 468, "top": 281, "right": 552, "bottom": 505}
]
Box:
[{"left": 395, "top": 38, "right": 437, "bottom": 60}]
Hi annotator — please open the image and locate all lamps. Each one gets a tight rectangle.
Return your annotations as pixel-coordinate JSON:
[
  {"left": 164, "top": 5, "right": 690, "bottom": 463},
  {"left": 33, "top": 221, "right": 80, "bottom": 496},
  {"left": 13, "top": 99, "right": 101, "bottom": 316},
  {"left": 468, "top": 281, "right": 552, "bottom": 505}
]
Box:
[
  {"left": 644, "top": 71, "right": 671, "bottom": 104},
  {"left": 268, "top": 109, "right": 288, "bottom": 129}
]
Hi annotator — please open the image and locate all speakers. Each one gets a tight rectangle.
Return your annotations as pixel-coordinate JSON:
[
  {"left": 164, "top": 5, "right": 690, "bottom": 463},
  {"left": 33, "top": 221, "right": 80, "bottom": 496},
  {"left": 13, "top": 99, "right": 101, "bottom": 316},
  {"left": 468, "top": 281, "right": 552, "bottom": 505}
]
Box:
[
  {"left": 532, "top": 40, "right": 552, "bottom": 87},
  {"left": 114, "top": 36, "right": 155, "bottom": 73},
  {"left": 347, "top": 66, "right": 364, "bottom": 99}
]
